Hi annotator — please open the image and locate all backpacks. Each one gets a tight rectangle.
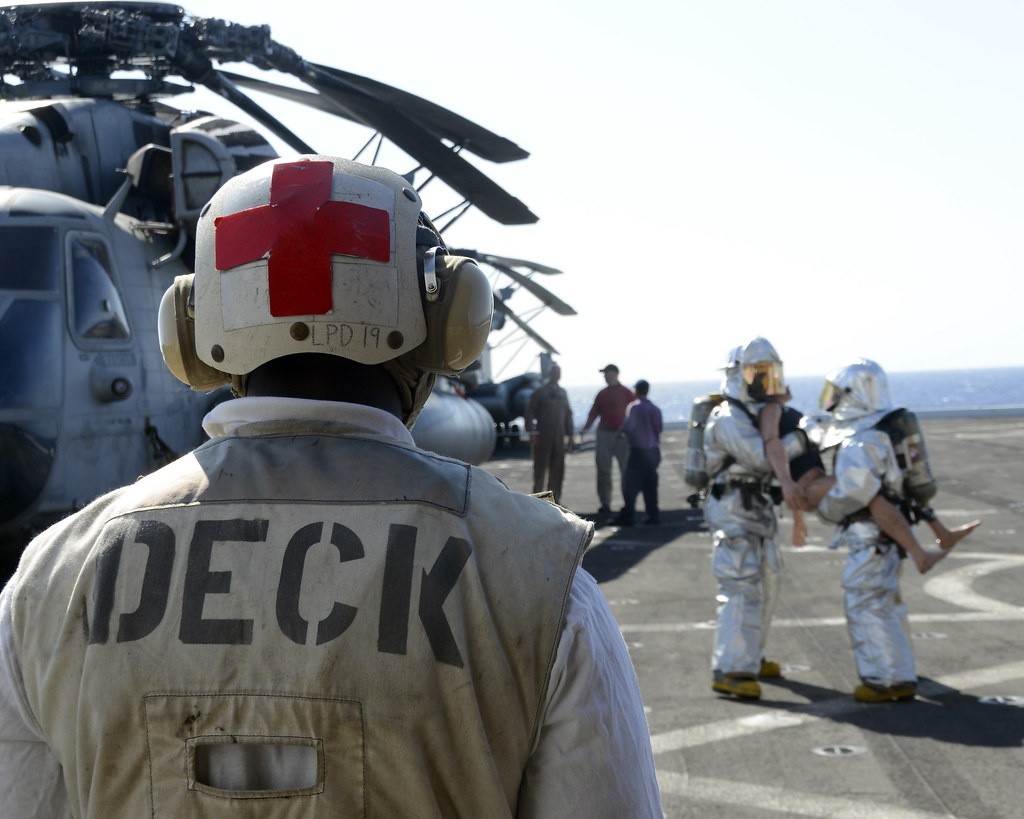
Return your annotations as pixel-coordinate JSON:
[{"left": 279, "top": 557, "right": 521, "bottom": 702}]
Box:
[
  {"left": 876, "top": 406, "right": 937, "bottom": 509},
  {"left": 683, "top": 393, "right": 726, "bottom": 486}
]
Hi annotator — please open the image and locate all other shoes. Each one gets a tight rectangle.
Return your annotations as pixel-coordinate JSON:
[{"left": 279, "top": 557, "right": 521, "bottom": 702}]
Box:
[
  {"left": 855, "top": 680, "right": 916, "bottom": 702},
  {"left": 759, "top": 659, "right": 780, "bottom": 678},
  {"left": 713, "top": 672, "right": 760, "bottom": 702}
]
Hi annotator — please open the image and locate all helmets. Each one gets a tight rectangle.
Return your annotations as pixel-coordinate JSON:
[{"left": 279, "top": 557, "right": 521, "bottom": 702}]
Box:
[
  {"left": 818, "top": 360, "right": 884, "bottom": 413},
  {"left": 722, "top": 334, "right": 784, "bottom": 395}
]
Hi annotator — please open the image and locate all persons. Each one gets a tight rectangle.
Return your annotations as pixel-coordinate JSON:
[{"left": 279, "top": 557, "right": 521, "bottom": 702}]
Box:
[
  {"left": 1, "top": 153, "right": 666, "bottom": 819},
  {"left": 703, "top": 338, "right": 806, "bottom": 702},
  {"left": 614, "top": 379, "right": 664, "bottom": 525},
  {"left": 763, "top": 383, "right": 981, "bottom": 573},
  {"left": 577, "top": 364, "right": 638, "bottom": 514},
  {"left": 811, "top": 358, "right": 941, "bottom": 702},
  {"left": 525, "top": 361, "right": 578, "bottom": 505}
]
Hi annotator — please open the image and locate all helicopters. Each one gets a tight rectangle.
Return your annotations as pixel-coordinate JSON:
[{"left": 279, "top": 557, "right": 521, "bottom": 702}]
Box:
[
  {"left": 2, "top": 2, "right": 540, "bottom": 535},
  {"left": 411, "top": 248, "right": 577, "bottom": 463}
]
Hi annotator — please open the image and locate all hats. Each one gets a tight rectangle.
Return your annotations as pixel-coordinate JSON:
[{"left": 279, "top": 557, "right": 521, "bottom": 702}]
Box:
[
  {"left": 600, "top": 364, "right": 619, "bottom": 373},
  {"left": 633, "top": 380, "right": 648, "bottom": 390},
  {"left": 191, "top": 155, "right": 444, "bottom": 379}
]
